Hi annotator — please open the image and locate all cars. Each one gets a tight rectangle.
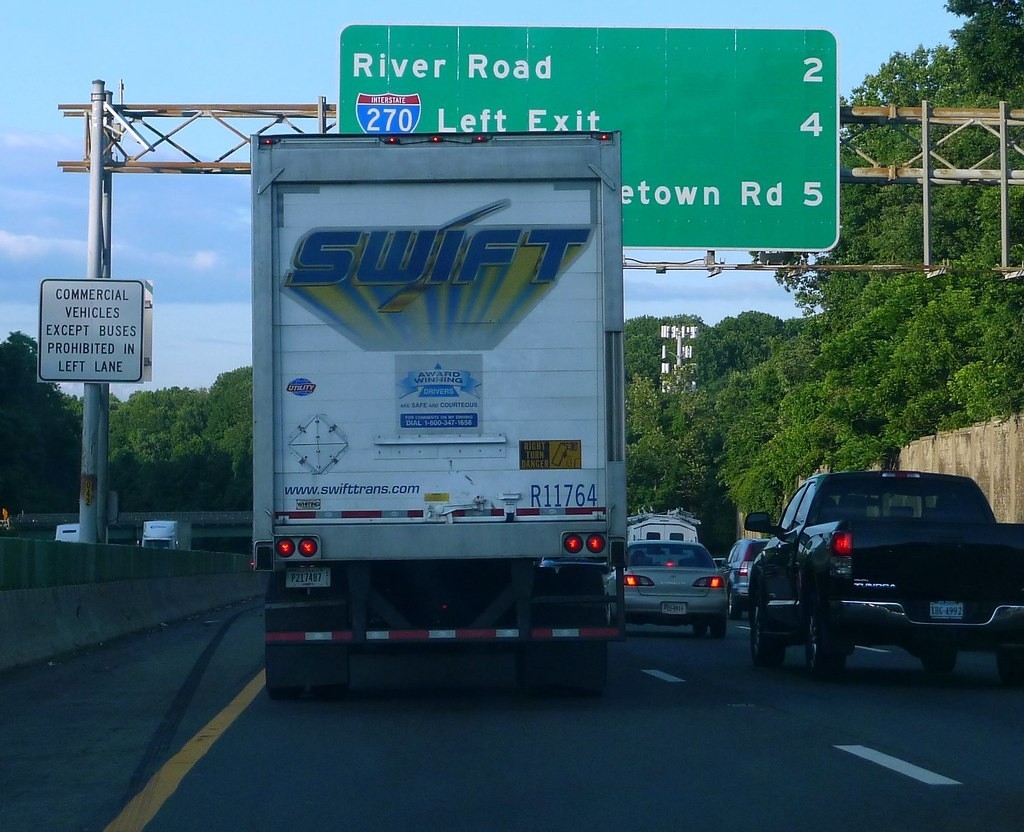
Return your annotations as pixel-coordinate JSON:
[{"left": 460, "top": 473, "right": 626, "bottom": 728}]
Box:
[
  {"left": 618, "top": 539, "right": 727, "bottom": 638},
  {"left": 716, "top": 537, "right": 775, "bottom": 619}
]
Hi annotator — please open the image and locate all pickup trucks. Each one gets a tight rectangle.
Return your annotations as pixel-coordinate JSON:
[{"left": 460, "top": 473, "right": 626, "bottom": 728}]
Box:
[{"left": 744, "top": 469, "right": 1024, "bottom": 690}]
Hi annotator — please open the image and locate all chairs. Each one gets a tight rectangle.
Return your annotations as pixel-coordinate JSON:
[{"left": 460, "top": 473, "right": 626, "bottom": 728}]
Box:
[
  {"left": 631, "top": 557, "right": 653, "bottom": 566},
  {"left": 678, "top": 558, "right": 705, "bottom": 567}
]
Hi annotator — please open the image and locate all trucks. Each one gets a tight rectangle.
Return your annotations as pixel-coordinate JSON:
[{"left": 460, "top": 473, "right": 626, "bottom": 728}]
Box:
[
  {"left": 137, "top": 520, "right": 192, "bottom": 552},
  {"left": 249, "top": 124, "right": 628, "bottom": 703}
]
